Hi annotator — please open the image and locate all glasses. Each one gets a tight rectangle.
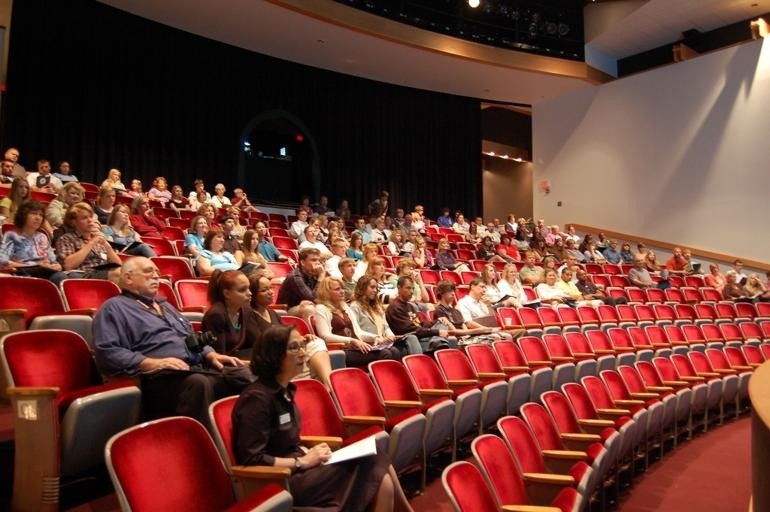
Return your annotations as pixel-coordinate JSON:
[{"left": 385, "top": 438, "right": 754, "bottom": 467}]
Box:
[
  {"left": 256, "top": 286, "right": 275, "bottom": 294},
  {"left": 287, "top": 341, "right": 307, "bottom": 353}
]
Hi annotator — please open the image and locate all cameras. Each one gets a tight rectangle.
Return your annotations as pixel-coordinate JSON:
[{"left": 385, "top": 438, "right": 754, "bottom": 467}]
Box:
[{"left": 185, "top": 330, "right": 219, "bottom": 353}]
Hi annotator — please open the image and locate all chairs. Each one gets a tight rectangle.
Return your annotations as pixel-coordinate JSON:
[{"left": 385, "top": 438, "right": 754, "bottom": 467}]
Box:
[{"left": 0, "top": 172, "right": 770, "bottom": 512}]
